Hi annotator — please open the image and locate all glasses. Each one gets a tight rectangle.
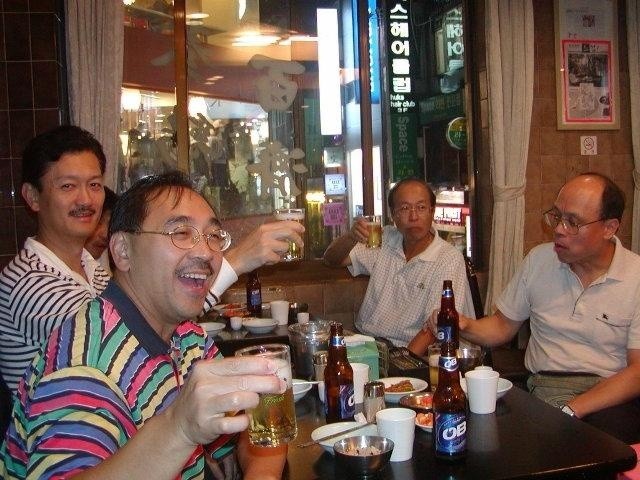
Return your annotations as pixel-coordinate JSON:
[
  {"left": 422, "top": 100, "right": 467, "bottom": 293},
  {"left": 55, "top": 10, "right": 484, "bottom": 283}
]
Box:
[
  {"left": 392, "top": 204, "right": 436, "bottom": 219},
  {"left": 126, "top": 225, "right": 232, "bottom": 251},
  {"left": 544, "top": 209, "right": 611, "bottom": 234}
]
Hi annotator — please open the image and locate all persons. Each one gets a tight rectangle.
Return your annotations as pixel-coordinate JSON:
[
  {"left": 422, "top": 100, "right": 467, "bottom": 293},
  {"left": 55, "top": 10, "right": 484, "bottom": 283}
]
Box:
[
  {"left": 84, "top": 185, "right": 121, "bottom": 260},
  {"left": 323, "top": 177, "right": 474, "bottom": 359},
  {"left": 0, "top": 170, "right": 285, "bottom": 480},
  {"left": 0, "top": 127, "right": 306, "bottom": 405},
  {"left": 423, "top": 171, "right": 640, "bottom": 445}
]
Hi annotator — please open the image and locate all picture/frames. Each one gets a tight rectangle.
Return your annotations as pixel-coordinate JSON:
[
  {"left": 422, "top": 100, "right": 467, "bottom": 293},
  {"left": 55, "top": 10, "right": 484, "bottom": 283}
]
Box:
[{"left": 553, "top": 0, "right": 621, "bottom": 129}]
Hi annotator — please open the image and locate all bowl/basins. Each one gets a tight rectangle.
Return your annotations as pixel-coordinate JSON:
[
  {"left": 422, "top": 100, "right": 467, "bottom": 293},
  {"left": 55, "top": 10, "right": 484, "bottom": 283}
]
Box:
[
  {"left": 460, "top": 378, "right": 514, "bottom": 401},
  {"left": 457, "top": 342, "right": 481, "bottom": 372},
  {"left": 293, "top": 378, "right": 312, "bottom": 403},
  {"left": 375, "top": 377, "right": 436, "bottom": 410},
  {"left": 311, "top": 421, "right": 393, "bottom": 475},
  {"left": 198, "top": 302, "right": 278, "bottom": 338},
  {"left": 290, "top": 302, "right": 308, "bottom": 322}
]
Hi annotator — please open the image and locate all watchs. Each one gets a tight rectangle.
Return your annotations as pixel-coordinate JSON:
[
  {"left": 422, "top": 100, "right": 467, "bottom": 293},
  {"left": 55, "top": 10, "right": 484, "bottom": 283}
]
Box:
[{"left": 559, "top": 404, "right": 579, "bottom": 420}]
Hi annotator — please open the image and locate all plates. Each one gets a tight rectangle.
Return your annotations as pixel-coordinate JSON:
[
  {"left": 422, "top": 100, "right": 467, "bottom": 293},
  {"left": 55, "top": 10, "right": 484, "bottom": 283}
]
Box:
[{"left": 416, "top": 414, "right": 432, "bottom": 432}]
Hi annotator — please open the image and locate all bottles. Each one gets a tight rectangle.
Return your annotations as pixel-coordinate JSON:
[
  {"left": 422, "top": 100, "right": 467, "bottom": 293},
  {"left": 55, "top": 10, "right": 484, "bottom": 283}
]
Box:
[
  {"left": 246, "top": 272, "right": 261, "bottom": 312},
  {"left": 433, "top": 279, "right": 469, "bottom": 462},
  {"left": 313, "top": 321, "right": 384, "bottom": 423}
]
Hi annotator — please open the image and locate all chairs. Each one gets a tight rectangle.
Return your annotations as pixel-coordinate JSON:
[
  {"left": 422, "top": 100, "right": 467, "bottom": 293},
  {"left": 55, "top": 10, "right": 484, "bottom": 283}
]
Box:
[
  {"left": 0, "top": 373, "right": 15, "bottom": 443},
  {"left": 463, "top": 246, "right": 533, "bottom": 392}
]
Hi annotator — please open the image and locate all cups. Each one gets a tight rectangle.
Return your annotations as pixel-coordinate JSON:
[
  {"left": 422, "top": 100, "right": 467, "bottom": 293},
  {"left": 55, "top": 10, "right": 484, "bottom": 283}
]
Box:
[
  {"left": 297, "top": 312, "right": 310, "bottom": 323},
  {"left": 365, "top": 216, "right": 382, "bottom": 248},
  {"left": 466, "top": 370, "right": 499, "bottom": 415},
  {"left": 236, "top": 344, "right": 298, "bottom": 447},
  {"left": 376, "top": 408, "right": 416, "bottom": 462},
  {"left": 428, "top": 343, "right": 441, "bottom": 391},
  {"left": 275, "top": 208, "right": 305, "bottom": 262},
  {"left": 271, "top": 301, "right": 289, "bottom": 325}
]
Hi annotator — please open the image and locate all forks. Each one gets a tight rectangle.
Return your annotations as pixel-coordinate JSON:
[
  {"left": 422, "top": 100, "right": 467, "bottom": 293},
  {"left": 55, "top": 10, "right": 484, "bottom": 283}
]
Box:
[{"left": 392, "top": 347, "right": 422, "bottom": 370}]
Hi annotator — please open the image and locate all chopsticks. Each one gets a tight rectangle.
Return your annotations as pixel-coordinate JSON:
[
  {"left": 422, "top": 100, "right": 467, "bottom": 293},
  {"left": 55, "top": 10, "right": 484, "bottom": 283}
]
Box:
[{"left": 299, "top": 422, "right": 373, "bottom": 449}]
